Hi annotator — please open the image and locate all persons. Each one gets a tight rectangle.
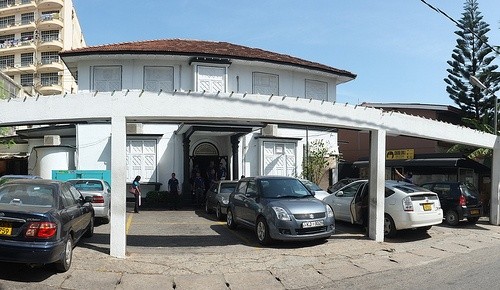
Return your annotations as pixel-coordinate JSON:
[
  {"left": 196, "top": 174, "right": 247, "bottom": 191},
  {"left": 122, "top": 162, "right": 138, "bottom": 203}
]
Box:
[
  {"left": 133, "top": 176, "right": 142, "bottom": 213},
  {"left": 395, "top": 168, "right": 414, "bottom": 184},
  {"left": 191, "top": 162, "right": 205, "bottom": 209},
  {"left": 206, "top": 158, "right": 226, "bottom": 188},
  {"left": 168, "top": 173, "right": 179, "bottom": 211}
]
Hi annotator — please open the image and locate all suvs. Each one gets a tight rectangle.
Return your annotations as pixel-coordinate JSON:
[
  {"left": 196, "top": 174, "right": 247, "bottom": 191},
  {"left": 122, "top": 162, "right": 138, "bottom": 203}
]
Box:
[
  {"left": 226, "top": 175, "right": 335, "bottom": 246},
  {"left": 419, "top": 182, "right": 483, "bottom": 226},
  {"left": 321, "top": 179, "right": 443, "bottom": 239}
]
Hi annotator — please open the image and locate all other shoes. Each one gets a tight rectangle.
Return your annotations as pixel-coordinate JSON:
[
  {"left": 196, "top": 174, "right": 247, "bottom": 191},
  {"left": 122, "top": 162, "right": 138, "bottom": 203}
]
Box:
[{"left": 134, "top": 211, "right": 138, "bottom": 213}]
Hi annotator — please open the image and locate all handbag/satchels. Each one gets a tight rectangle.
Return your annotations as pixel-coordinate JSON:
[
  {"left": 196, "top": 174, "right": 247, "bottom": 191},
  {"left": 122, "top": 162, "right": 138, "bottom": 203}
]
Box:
[{"left": 129, "top": 185, "right": 136, "bottom": 194}]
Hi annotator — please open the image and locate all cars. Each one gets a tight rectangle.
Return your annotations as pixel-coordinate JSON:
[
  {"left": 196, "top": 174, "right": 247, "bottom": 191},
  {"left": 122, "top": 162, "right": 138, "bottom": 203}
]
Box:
[
  {"left": 65, "top": 178, "right": 111, "bottom": 224},
  {"left": 0, "top": 175, "right": 43, "bottom": 184},
  {"left": 296, "top": 178, "right": 331, "bottom": 202},
  {"left": 326, "top": 177, "right": 367, "bottom": 194},
  {"left": 204, "top": 180, "right": 239, "bottom": 219},
  {"left": 0, "top": 179, "right": 96, "bottom": 272}
]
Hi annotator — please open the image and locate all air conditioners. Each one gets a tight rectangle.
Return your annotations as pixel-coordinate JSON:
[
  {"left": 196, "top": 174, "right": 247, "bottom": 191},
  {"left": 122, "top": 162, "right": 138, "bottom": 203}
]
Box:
[
  {"left": 261, "top": 124, "right": 278, "bottom": 136},
  {"left": 127, "top": 123, "right": 143, "bottom": 134},
  {"left": 44, "top": 135, "right": 61, "bottom": 145}
]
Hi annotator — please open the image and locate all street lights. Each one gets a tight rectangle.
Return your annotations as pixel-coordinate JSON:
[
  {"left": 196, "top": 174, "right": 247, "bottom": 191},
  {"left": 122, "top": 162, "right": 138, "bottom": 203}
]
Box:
[{"left": 469, "top": 74, "right": 498, "bottom": 134}]
